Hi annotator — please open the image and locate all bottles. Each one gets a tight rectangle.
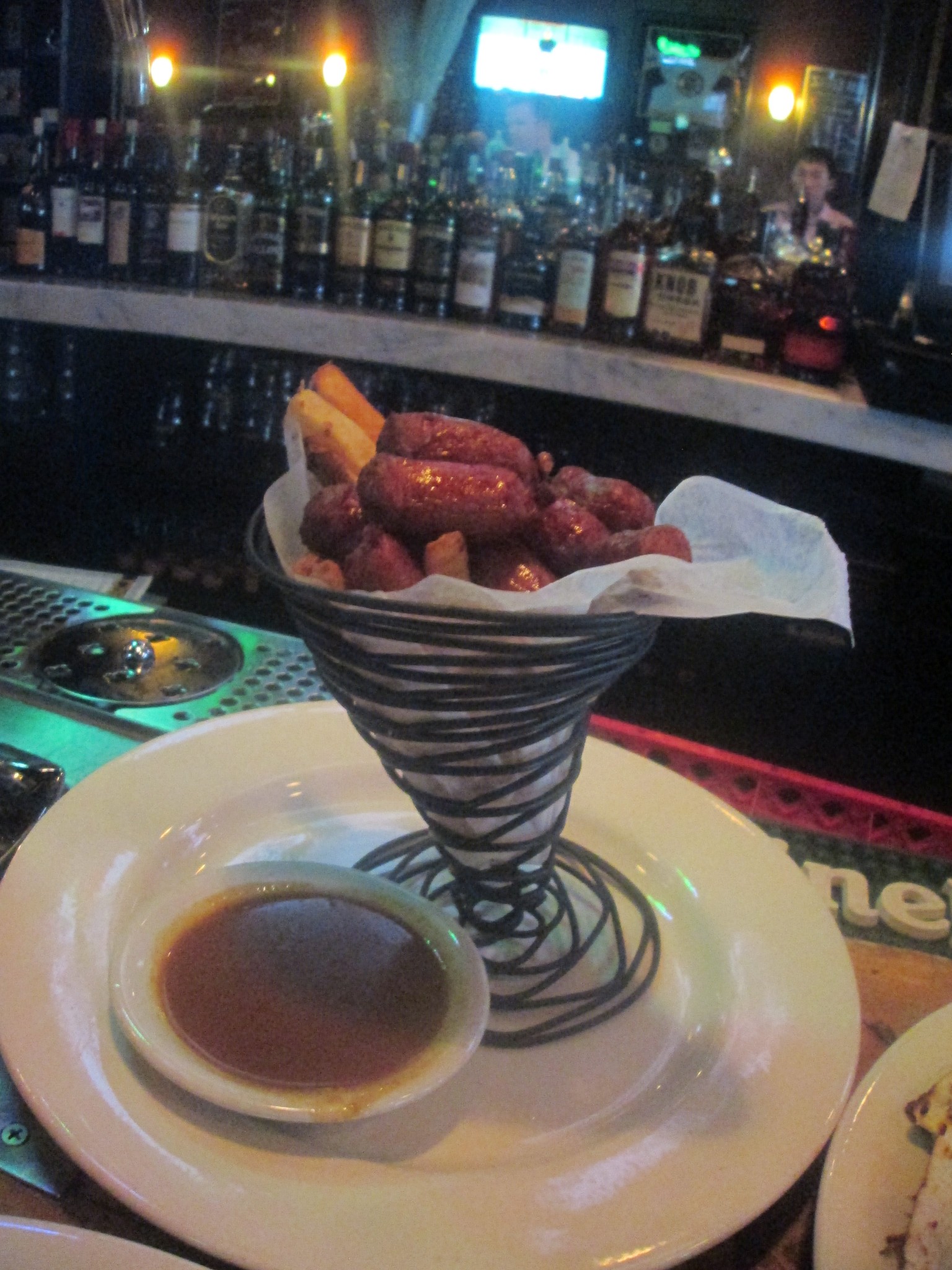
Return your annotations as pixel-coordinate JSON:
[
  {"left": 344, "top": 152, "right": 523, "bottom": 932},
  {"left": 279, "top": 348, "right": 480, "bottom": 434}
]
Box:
[
  {"left": 287, "top": 117, "right": 339, "bottom": 302},
  {"left": 545, "top": 156, "right": 629, "bottom": 338},
  {"left": 639, "top": 169, "right": 767, "bottom": 356},
  {"left": 593, "top": 168, "right": 681, "bottom": 345},
  {"left": 496, "top": 156, "right": 577, "bottom": 330},
  {"left": 104, "top": 117, "right": 142, "bottom": 281},
  {"left": 18, "top": 107, "right": 85, "bottom": 278},
  {"left": 769, "top": 199, "right": 853, "bottom": 387},
  {"left": 133, "top": 123, "right": 174, "bottom": 283},
  {"left": 205, "top": 145, "right": 254, "bottom": 290},
  {"left": 339, "top": 138, "right": 372, "bottom": 305},
  {"left": 76, "top": 117, "right": 114, "bottom": 276},
  {"left": 451, "top": 148, "right": 524, "bottom": 322},
  {"left": 254, "top": 148, "right": 292, "bottom": 291},
  {"left": 165, "top": 118, "right": 204, "bottom": 287},
  {"left": 373, "top": 121, "right": 459, "bottom": 319}
]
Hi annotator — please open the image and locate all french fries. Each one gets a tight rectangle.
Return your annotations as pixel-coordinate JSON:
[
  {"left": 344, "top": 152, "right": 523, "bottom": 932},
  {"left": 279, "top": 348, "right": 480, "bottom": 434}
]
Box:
[{"left": 285, "top": 363, "right": 387, "bottom": 484}]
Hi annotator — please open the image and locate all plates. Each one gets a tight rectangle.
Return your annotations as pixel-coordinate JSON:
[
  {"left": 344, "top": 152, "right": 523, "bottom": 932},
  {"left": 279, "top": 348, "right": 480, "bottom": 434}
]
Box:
[
  {"left": 0, "top": 1214, "right": 211, "bottom": 1270},
  {"left": 813, "top": 1001, "right": 952, "bottom": 1270},
  {"left": 0, "top": 699, "right": 951, "bottom": 1270}
]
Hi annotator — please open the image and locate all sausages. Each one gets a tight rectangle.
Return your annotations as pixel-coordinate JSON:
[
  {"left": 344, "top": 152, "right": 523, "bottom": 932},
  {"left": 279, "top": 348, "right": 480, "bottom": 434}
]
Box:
[{"left": 294, "top": 413, "right": 692, "bottom": 598}]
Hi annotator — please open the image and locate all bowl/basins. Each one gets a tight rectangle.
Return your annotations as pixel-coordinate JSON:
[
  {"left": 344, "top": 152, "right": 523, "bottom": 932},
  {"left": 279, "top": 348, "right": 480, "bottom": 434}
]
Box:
[{"left": 112, "top": 859, "right": 492, "bottom": 1124}]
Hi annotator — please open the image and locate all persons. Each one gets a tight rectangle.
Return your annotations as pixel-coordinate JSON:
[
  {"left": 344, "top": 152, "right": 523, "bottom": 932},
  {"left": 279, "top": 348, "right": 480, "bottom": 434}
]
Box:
[
  {"left": 465, "top": 92, "right": 586, "bottom": 204},
  {"left": 754, "top": 146, "right": 855, "bottom": 270}
]
